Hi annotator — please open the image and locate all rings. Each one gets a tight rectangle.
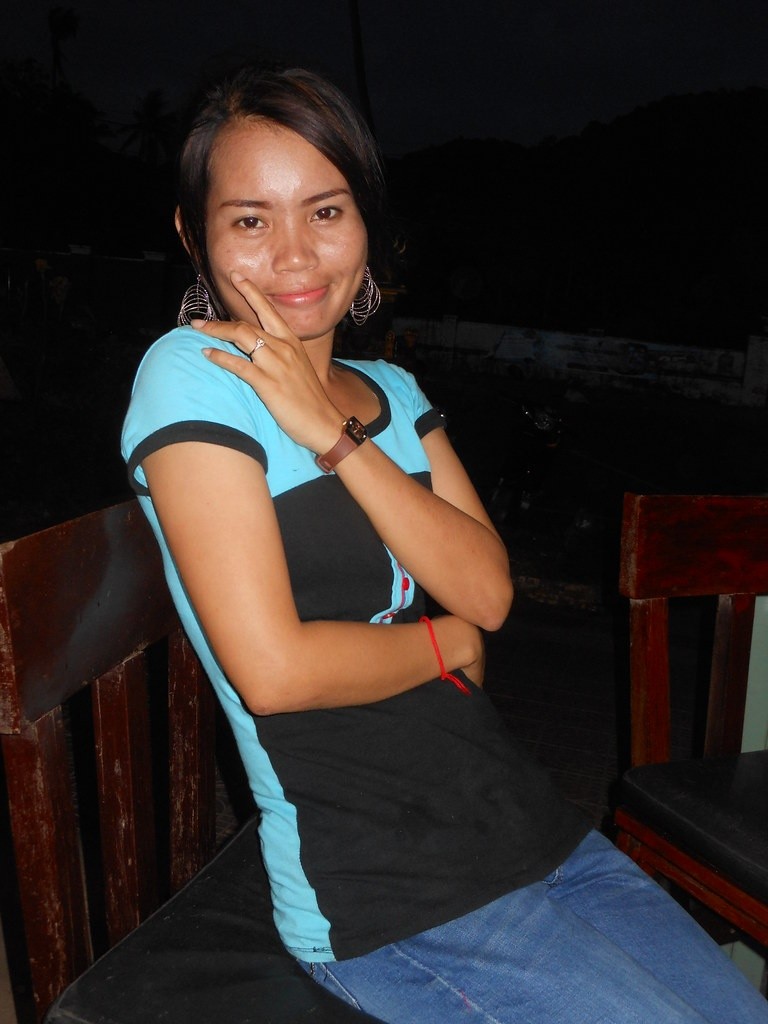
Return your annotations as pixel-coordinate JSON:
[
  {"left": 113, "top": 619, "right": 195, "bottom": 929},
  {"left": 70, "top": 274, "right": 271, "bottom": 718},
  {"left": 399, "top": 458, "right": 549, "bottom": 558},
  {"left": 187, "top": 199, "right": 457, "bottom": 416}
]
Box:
[{"left": 248, "top": 339, "right": 265, "bottom": 357}]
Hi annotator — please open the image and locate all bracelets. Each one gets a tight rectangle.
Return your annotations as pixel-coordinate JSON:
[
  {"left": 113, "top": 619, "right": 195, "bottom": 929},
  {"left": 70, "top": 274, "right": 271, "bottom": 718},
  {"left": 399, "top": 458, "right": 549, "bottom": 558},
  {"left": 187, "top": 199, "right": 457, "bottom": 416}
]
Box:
[{"left": 419, "top": 616, "right": 471, "bottom": 695}]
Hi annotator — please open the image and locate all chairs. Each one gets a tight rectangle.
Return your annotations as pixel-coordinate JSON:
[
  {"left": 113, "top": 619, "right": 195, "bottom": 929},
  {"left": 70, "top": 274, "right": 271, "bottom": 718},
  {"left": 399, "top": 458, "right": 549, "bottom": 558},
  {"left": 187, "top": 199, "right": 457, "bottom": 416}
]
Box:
[
  {"left": 615, "top": 490, "right": 768, "bottom": 953},
  {"left": 1, "top": 497, "right": 385, "bottom": 1024}
]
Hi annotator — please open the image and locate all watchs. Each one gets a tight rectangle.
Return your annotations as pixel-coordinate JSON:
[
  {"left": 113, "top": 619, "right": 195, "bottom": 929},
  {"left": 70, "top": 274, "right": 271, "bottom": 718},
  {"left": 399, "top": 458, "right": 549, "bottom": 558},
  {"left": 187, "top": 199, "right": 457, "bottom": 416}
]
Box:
[{"left": 316, "top": 416, "right": 368, "bottom": 475}]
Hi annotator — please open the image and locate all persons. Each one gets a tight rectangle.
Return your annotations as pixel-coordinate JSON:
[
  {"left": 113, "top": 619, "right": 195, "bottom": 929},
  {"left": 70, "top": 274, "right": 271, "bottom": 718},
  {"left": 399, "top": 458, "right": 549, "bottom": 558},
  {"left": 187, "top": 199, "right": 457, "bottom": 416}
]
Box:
[{"left": 120, "top": 67, "right": 768, "bottom": 1024}]
